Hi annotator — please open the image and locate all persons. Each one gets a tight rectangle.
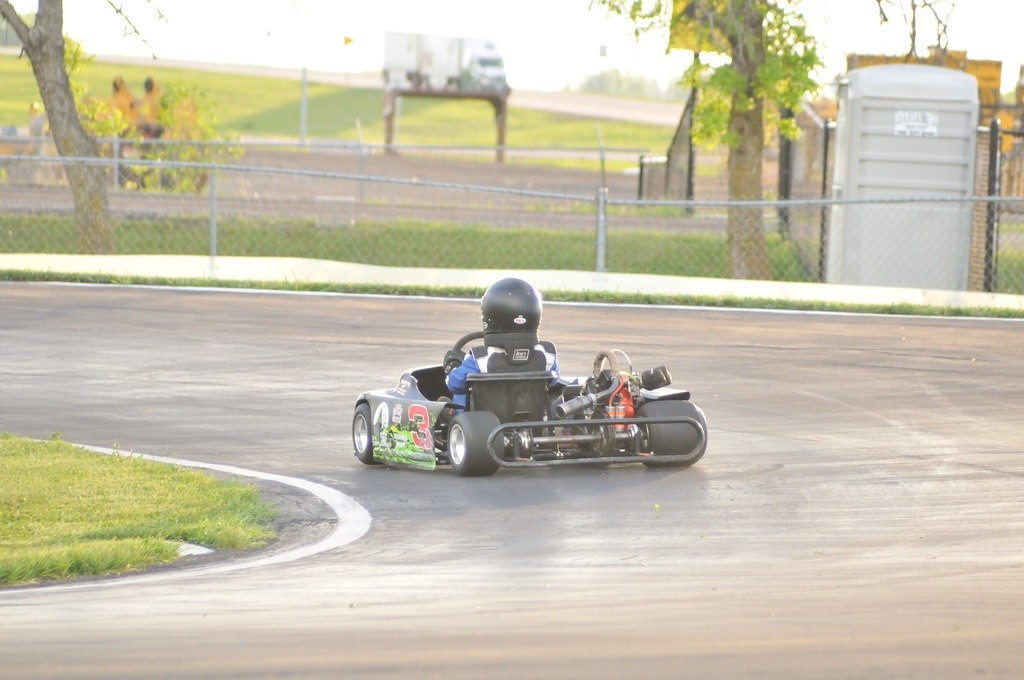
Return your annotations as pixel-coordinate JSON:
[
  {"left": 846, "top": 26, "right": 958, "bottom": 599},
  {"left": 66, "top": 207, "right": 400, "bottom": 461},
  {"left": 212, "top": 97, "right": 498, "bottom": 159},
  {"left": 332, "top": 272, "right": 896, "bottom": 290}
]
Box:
[{"left": 437, "top": 278, "right": 559, "bottom": 424}]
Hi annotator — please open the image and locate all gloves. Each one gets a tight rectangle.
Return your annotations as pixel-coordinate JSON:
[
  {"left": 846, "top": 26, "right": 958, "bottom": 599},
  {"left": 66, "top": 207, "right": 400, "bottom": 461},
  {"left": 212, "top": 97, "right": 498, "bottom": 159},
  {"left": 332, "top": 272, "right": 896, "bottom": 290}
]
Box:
[{"left": 443, "top": 349, "right": 465, "bottom": 375}]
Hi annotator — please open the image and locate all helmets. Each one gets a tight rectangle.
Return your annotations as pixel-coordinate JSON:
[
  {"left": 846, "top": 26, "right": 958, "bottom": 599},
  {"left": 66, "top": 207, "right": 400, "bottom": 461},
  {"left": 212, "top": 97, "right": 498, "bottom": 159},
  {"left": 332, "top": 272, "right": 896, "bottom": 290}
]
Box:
[{"left": 479, "top": 278, "right": 541, "bottom": 334}]
